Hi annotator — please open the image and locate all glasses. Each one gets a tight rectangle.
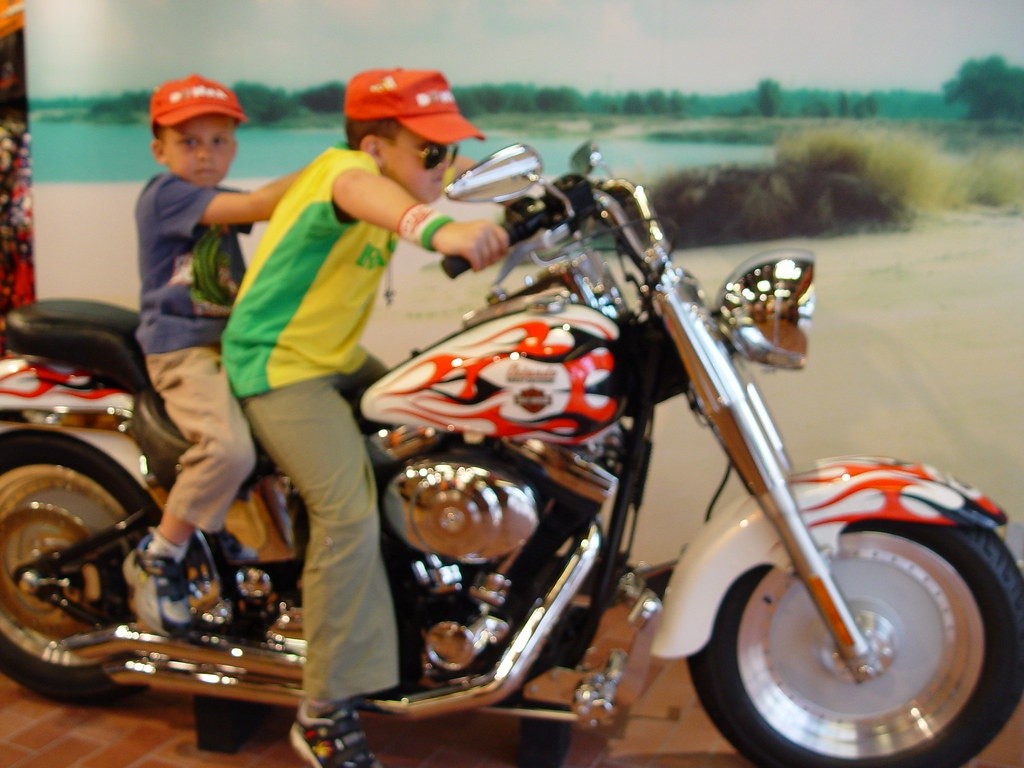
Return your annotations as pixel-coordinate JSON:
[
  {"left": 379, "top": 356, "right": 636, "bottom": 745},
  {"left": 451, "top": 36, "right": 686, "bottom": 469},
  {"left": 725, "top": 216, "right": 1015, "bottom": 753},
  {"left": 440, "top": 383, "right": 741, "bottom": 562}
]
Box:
[{"left": 378, "top": 137, "right": 458, "bottom": 169}]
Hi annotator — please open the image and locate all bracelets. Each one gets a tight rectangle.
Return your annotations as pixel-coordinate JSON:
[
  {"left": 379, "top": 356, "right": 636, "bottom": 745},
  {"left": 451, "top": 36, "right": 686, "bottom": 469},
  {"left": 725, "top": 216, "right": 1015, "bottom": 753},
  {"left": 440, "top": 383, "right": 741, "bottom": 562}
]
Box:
[{"left": 397, "top": 201, "right": 457, "bottom": 252}]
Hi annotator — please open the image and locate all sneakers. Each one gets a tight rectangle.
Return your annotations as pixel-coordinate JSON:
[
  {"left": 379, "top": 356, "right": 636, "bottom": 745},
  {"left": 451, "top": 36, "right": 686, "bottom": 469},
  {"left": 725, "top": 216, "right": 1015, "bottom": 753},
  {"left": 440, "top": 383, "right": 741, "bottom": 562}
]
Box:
[
  {"left": 290, "top": 700, "right": 382, "bottom": 768},
  {"left": 123, "top": 534, "right": 193, "bottom": 638}
]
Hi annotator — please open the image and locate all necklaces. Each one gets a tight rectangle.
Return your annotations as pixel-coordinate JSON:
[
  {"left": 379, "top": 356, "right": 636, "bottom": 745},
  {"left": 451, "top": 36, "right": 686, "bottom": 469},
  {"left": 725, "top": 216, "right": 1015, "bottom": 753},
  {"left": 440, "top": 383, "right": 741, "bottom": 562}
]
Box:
[{"left": 383, "top": 226, "right": 397, "bottom": 304}]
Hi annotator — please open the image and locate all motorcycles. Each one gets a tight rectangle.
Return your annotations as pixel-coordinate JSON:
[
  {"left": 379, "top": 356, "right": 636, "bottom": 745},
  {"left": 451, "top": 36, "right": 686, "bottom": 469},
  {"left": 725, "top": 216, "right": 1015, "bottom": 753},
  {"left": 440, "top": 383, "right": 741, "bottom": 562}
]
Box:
[{"left": 1, "top": 135, "right": 1024, "bottom": 767}]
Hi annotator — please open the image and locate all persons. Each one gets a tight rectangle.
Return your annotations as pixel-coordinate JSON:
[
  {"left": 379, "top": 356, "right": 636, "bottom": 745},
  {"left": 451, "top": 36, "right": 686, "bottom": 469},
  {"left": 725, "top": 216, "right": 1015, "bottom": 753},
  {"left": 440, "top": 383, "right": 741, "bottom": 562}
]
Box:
[
  {"left": 121, "top": 76, "right": 314, "bottom": 636},
  {"left": 222, "top": 71, "right": 508, "bottom": 768}
]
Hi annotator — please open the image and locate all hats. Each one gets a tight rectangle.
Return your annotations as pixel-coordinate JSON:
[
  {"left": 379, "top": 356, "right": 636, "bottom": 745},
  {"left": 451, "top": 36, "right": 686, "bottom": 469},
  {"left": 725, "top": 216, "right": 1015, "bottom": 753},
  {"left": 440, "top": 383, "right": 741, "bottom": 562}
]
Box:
[
  {"left": 150, "top": 73, "right": 248, "bottom": 133},
  {"left": 345, "top": 67, "right": 484, "bottom": 144}
]
